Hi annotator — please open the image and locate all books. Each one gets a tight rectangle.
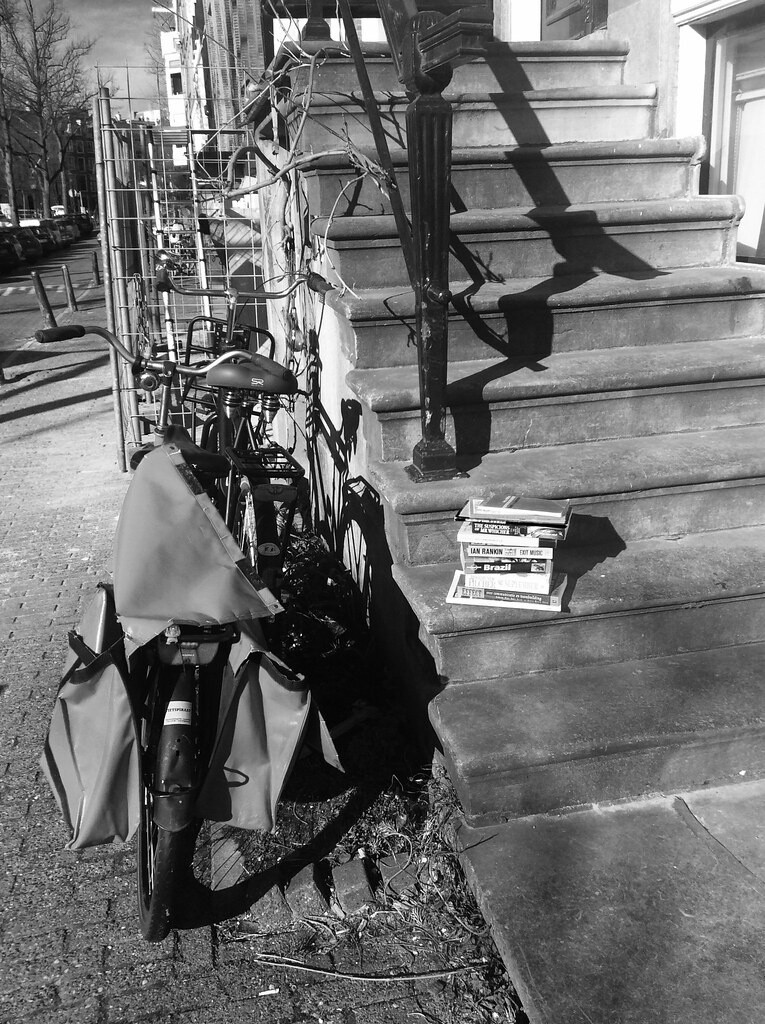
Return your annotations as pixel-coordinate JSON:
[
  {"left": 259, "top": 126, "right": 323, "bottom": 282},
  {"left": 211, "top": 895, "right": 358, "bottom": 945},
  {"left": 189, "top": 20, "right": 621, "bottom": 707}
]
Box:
[{"left": 446, "top": 492, "right": 573, "bottom": 612}]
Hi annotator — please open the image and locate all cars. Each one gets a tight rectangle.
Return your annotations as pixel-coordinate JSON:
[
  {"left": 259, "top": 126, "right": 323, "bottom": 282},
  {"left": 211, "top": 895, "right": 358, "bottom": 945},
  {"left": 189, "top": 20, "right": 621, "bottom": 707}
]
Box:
[{"left": 1, "top": 211, "right": 96, "bottom": 273}]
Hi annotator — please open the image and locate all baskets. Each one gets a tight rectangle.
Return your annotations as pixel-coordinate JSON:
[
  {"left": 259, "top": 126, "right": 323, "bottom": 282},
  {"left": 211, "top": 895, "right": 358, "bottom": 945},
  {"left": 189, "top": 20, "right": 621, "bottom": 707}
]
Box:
[{"left": 178, "top": 315, "right": 275, "bottom": 420}]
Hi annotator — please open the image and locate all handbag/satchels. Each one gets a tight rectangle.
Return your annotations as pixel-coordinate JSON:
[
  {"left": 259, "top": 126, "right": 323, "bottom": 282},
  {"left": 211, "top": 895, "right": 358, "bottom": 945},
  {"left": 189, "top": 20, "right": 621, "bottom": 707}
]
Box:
[
  {"left": 39, "top": 443, "right": 285, "bottom": 856},
  {"left": 209, "top": 619, "right": 348, "bottom": 837}
]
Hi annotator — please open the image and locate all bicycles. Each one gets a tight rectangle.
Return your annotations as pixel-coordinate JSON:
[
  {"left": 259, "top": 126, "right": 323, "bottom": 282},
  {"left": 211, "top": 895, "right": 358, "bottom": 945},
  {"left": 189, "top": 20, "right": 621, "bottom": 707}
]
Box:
[{"left": 28, "top": 318, "right": 320, "bottom": 946}]
